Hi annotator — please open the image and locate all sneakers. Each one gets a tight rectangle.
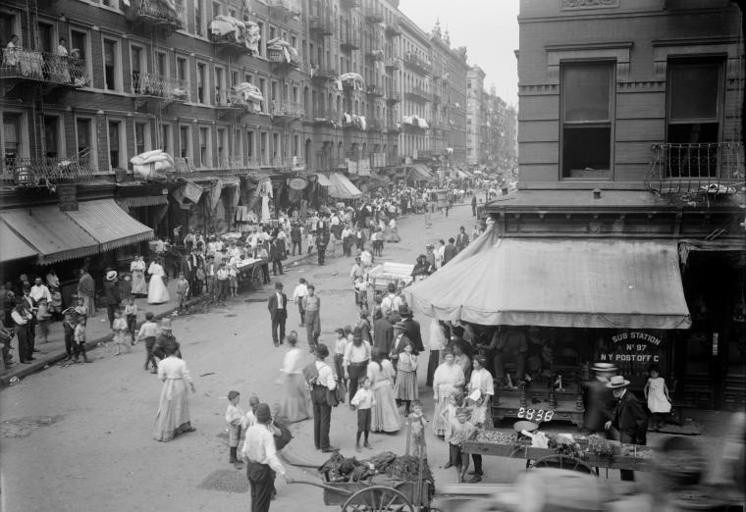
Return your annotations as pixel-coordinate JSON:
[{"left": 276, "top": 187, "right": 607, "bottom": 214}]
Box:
[{"left": 391, "top": 321, "right": 408, "bottom": 330}]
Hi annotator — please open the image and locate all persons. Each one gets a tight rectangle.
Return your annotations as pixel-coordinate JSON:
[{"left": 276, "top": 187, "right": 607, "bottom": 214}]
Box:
[
  {"left": 224, "top": 219, "right": 673, "bottom": 512},
  {"left": 173, "top": 164, "right": 520, "bottom": 305},
  {"left": 1, "top": 236, "right": 196, "bottom": 439}
]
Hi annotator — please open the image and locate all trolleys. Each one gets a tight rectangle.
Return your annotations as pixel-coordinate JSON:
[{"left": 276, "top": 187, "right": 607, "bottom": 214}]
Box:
[{"left": 282, "top": 452, "right": 435, "bottom": 512}]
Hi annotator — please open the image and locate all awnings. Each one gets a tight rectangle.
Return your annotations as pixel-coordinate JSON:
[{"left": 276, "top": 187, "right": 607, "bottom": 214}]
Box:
[{"left": 1, "top": 199, "right": 155, "bottom": 268}]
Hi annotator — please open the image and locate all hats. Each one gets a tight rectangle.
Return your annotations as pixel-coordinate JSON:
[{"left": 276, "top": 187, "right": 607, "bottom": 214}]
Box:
[
  {"left": 605, "top": 375, "right": 631, "bottom": 389},
  {"left": 590, "top": 361, "right": 619, "bottom": 372},
  {"left": 106, "top": 270, "right": 118, "bottom": 281},
  {"left": 275, "top": 282, "right": 284, "bottom": 289},
  {"left": 313, "top": 343, "right": 329, "bottom": 358},
  {"left": 512, "top": 419, "right": 540, "bottom": 435},
  {"left": 393, "top": 304, "right": 412, "bottom": 316}
]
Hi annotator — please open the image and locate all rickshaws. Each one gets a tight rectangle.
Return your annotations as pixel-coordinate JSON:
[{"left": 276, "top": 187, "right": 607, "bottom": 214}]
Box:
[
  {"left": 459, "top": 428, "right": 707, "bottom": 481},
  {"left": 233, "top": 257, "right": 265, "bottom": 290}
]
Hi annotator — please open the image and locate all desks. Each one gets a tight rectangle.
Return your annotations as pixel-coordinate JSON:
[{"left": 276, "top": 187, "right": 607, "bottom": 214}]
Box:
[{"left": 491, "top": 395, "right": 586, "bottom": 433}]
